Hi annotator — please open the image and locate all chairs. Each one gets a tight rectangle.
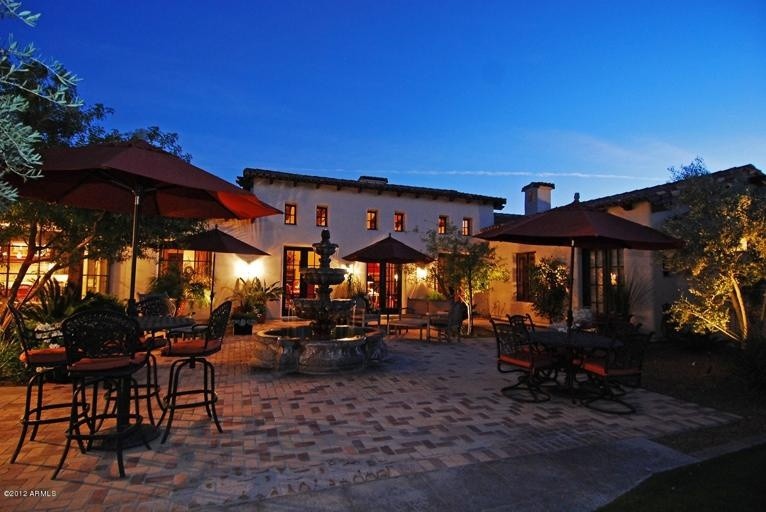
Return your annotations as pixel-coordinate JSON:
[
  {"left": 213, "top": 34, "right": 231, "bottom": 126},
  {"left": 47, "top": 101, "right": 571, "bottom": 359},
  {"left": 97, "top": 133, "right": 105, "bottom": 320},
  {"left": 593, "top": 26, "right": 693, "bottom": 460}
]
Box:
[
  {"left": 488, "top": 311, "right": 644, "bottom": 405},
  {"left": 378, "top": 297, "right": 461, "bottom": 345},
  {"left": 5, "top": 291, "right": 232, "bottom": 482}
]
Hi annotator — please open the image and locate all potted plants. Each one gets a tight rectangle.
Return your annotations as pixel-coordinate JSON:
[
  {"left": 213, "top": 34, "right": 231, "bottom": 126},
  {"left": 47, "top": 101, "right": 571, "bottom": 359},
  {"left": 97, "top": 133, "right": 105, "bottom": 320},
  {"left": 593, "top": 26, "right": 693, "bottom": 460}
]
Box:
[{"left": 220, "top": 276, "right": 284, "bottom": 335}]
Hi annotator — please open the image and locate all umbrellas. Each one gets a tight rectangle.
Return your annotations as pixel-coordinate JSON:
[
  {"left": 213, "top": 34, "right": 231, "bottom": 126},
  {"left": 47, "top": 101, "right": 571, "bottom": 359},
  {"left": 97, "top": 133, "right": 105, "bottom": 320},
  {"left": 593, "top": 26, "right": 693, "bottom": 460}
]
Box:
[
  {"left": 340, "top": 232, "right": 436, "bottom": 334},
  {"left": 0, "top": 134, "right": 285, "bottom": 299},
  {"left": 473, "top": 192, "right": 688, "bottom": 336},
  {"left": 148, "top": 223, "right": 272, "bottom": 311}
]
